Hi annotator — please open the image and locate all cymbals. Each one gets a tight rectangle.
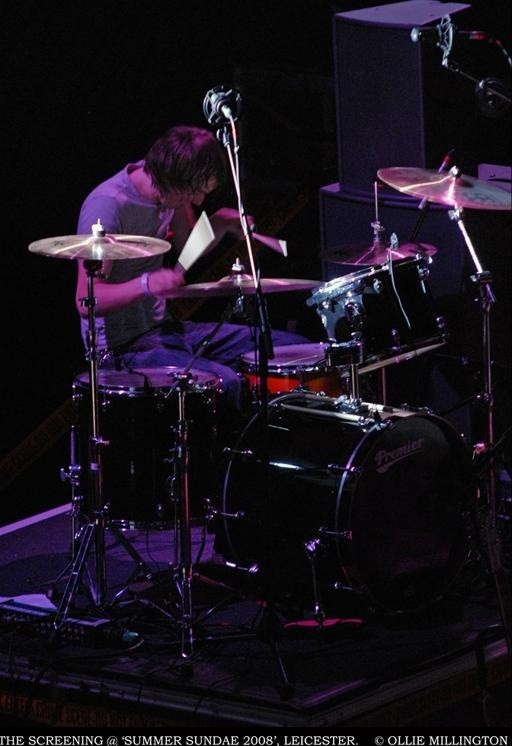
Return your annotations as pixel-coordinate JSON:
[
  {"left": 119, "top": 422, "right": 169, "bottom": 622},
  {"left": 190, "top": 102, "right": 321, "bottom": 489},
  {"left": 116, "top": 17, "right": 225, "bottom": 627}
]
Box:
[
  {"left": 26, "top": 233, "right": 173, "bottom": 263},
  {"left": 158, "top": 274, "right": 323, "bottom": 302},
  {"left": 328, "top": 240, "right": 440, "bottom": 267},
  {"left": 377, "top": 164, "right": 512, "bottom": 213}
]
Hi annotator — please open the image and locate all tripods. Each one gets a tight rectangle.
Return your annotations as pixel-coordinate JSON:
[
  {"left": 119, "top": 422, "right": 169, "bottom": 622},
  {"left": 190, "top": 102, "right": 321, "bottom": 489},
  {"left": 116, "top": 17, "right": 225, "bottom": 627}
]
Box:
[{"left": 29, "top": 263, "right": 263, "bottom": 699}]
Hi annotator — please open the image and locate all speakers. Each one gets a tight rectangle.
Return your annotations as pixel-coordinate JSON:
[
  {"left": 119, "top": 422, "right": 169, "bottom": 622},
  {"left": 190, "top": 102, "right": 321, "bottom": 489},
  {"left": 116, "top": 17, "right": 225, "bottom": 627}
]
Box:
[
  {"left": 332, "top": 0, "right": 479, "bottom": 202},
  {"left": 316, "top": 163, "right": 512, "bottom": 446}
]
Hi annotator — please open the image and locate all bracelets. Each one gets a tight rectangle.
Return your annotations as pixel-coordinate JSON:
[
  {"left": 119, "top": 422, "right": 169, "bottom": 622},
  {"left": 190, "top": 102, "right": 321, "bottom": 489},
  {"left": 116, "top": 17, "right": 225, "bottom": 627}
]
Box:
[{"left": 142, "top": 272, "right": 152, "bottom": 297}]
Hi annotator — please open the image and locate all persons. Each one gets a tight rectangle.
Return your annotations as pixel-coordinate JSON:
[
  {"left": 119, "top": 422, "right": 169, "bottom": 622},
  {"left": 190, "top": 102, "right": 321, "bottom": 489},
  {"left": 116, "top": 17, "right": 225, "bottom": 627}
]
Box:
[{"left": 76, "top": 126, "right": 314, "bottom": 408}]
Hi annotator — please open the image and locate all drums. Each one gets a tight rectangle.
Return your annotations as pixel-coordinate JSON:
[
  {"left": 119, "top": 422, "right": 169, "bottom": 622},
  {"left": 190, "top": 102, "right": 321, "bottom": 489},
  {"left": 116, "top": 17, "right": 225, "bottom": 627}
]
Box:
[
  {"left": 240, "top": 343, "right": 350, "bottom": 400},
  {"left": 65, "top": 362, "right": 247, "bottom": 537},
  {"left": 307, "top": 257, "right": 451, "bottom": 380},
  {"left": 207, "top": 389, "right": 488, "bottom": 650}
]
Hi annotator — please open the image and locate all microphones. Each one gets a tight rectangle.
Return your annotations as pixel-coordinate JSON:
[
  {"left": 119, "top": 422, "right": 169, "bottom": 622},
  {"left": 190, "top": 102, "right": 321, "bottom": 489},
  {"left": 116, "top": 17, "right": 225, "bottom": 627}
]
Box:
[
  {"left": 411, "top": 23, "right": 488, "bottom": 43},
  {"left": 203, "top": 86, "right": 244, "bottom": 124},
  {"left": 418, "top": 147, "right": 457, "bottom": 209},
  {"left": 261, "top": 294, "right": 277, "bottom": 361}
]
